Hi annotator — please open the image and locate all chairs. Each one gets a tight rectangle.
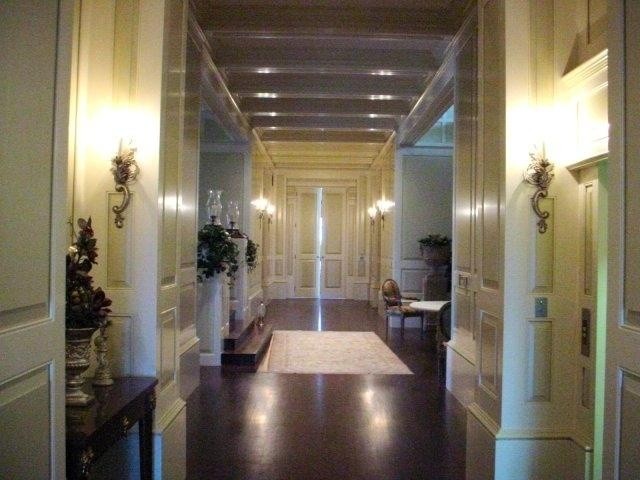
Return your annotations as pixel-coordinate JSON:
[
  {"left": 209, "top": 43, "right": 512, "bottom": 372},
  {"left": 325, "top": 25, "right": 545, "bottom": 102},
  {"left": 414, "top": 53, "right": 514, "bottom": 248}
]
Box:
[{"left": 383, "top": 279, "right": 424, "bottom": 341}]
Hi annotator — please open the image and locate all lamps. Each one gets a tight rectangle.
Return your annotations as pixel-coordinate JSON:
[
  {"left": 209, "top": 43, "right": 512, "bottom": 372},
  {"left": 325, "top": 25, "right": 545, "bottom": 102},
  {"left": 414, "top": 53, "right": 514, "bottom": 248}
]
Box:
[{"left": 205, "top": 190, "right": 241, "bottom": 230}]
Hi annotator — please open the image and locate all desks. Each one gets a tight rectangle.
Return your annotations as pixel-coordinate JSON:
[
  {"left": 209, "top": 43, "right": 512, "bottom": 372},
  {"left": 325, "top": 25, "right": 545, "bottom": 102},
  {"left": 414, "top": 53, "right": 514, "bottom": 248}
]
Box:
[
  {"left": 409, "top": 300, "right": 450, "bottom": 341},
  {"left": 67, "top": 376, "right": 158, "bottom": 478}
]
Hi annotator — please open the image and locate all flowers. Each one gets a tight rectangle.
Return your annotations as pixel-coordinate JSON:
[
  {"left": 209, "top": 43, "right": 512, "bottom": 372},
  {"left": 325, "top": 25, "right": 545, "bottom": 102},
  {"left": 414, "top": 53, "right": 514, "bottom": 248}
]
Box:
[{"left": 65, "top": 218, "right": 111, "bottom": 331}]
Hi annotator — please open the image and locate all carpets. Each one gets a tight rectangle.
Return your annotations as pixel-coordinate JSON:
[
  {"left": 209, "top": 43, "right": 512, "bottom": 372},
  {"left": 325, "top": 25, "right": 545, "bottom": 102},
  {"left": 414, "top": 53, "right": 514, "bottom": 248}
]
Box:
[{"left": 268, "top": 329, "right": 414, "bottom": 374}]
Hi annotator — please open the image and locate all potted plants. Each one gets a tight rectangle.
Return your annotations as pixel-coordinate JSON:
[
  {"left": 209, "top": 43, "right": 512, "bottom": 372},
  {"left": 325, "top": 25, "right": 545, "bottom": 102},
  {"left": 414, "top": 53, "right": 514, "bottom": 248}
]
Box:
[{"left": 419, "top": 232, "right": 449, "bottom": 266}]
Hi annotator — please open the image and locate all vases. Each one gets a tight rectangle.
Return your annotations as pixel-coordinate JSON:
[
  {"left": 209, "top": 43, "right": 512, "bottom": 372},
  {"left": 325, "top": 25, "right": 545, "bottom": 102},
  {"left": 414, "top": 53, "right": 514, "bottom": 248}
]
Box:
[{"left": 67, "top": 327, "right": 94, "bottom": 409}]
